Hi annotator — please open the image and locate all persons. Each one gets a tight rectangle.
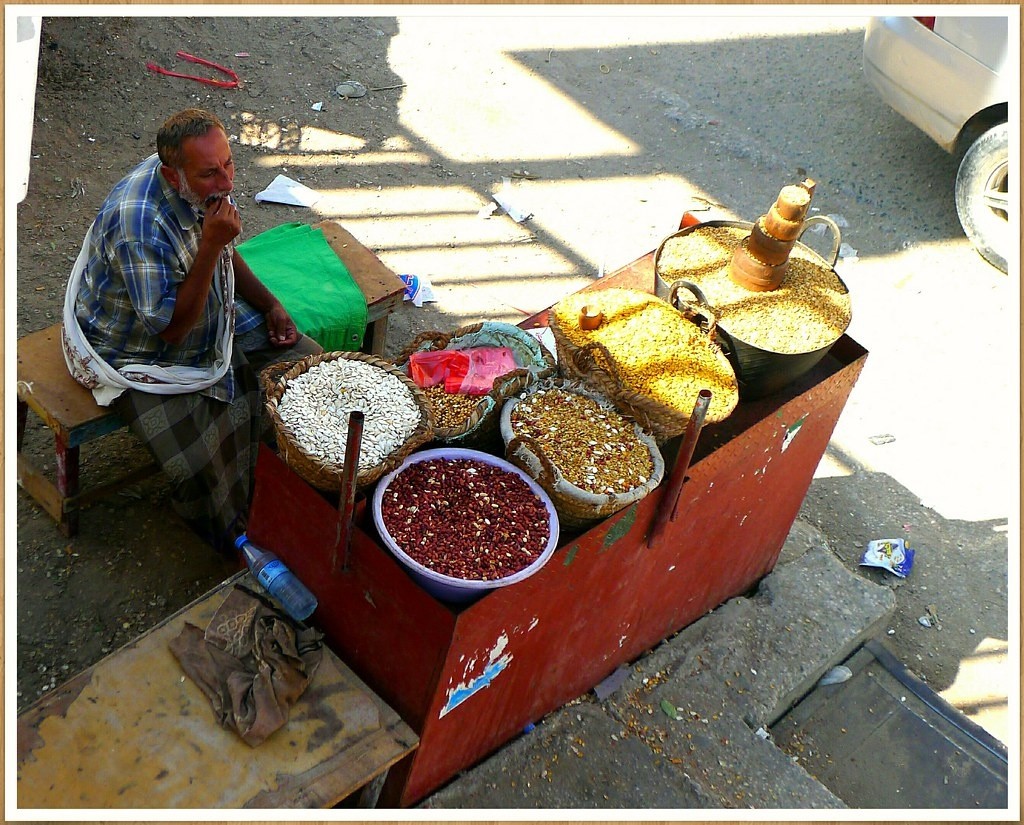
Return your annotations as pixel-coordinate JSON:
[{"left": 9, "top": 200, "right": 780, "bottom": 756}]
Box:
[{"left": 59, "top": 106, "right": 325, "bottom": 546}]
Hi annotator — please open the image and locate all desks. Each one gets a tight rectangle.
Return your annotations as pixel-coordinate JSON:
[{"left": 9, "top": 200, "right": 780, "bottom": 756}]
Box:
[{"left": 19, "top": 571, "right": 424, "bottom": 810}]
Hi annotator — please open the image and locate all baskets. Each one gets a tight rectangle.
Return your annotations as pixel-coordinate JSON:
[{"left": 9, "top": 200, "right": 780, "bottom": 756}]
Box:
[
  {"left": 386, "top": 321, "right": 558, "bottom": 452},
  {"left": 260, "top": 349, "right": 435, "bottom": 497},
  {"left": 500, "top": 376, "right": 666, "bottom": 536},
  {"left": 548, "top": 285, "right": 740, "bottom": 448}
]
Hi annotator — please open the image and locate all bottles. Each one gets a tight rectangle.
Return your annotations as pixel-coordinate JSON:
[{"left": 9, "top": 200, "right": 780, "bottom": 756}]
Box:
[{"left": 234, "top": 534, "right": 318, "bottom": 623}]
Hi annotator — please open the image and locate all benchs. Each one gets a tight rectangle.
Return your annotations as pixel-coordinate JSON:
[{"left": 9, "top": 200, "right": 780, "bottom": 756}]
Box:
[{"left": 18, "top": 217, "right": 407, "bottom": 537}]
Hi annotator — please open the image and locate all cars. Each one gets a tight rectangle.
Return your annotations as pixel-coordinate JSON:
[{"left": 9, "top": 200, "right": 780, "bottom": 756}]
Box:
[{"left": 862, "top": 16, "right": 1008, "bottom": 279}]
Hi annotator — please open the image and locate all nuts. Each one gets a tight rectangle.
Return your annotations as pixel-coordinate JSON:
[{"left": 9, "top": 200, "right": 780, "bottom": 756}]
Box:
[
  {"left": 381, "top": 456, "right": 550, "bottom": 582},
  {"left": 418, "top": 380, "right": 484, "bottom": 430},
  {"left": 657, "top": 225, "right": 851, "bottom": 353},
  {"left": 555, "top": 290, "right": 736, "bottom": 417},
  {"left": 511, "top": 388, "right": 655, "bottom": 495},
  {"left": 278, "top": 358, "right": 421, "bottom": 473}
]
